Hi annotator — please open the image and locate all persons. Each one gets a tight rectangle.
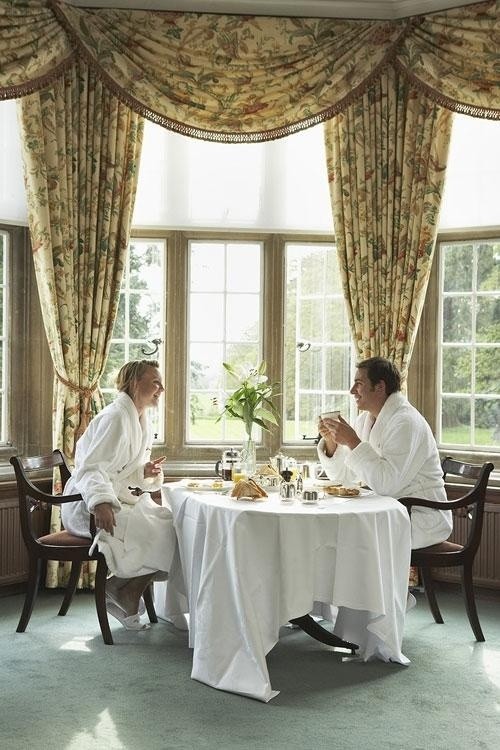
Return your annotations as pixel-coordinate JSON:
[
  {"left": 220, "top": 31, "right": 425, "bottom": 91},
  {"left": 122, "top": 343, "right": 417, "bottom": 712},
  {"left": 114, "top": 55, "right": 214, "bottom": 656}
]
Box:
[
  {"left": 61, "top": 358, "right": 176, "bottom": 631},
  {"left": 317, "top": 356, "right": 453, "bottom": 550}
]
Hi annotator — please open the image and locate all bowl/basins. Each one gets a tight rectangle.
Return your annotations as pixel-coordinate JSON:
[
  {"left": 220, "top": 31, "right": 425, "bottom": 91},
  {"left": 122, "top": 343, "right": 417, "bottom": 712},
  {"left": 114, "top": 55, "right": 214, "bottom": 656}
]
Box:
[{"left": 320, "top": 410, "right": 340, "bottom": 421}]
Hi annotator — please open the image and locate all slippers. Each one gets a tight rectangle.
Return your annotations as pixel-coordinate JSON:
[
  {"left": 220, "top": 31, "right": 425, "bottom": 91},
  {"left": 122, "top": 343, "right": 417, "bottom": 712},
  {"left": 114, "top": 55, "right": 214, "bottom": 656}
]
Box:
[{"left": 90, "top": 576, "right": 152, "bottom": 632}]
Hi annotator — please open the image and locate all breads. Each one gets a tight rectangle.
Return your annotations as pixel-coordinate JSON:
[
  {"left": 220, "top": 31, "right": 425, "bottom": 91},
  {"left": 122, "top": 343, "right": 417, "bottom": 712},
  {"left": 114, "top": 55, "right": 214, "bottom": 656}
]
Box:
[{"left": 231, "top": 479, "right": 268, "bottom": 498}]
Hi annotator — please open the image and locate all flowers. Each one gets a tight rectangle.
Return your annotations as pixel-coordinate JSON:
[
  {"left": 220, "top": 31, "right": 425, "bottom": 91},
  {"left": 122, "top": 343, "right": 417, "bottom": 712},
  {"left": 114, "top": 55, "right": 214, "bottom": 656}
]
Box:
[{"left": 211, "top": 350, "right": 281, "bottom": 445}]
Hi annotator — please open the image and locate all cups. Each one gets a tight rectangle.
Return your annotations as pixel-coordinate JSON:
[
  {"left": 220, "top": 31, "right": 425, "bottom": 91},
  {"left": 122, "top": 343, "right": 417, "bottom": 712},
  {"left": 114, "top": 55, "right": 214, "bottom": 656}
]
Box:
[{"left": 215, "top": 447, "right": 241, "bottom": 481}]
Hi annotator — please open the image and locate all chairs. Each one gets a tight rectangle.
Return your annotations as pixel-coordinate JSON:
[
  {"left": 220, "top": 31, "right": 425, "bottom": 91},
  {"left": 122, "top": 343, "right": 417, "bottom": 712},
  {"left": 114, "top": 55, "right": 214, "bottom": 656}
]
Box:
[
  {"left": 7, "top": 448, "right": 162, "bottom": 649},
  {"left": 389, "top": 453, "right": 496, "bottom": 645}
]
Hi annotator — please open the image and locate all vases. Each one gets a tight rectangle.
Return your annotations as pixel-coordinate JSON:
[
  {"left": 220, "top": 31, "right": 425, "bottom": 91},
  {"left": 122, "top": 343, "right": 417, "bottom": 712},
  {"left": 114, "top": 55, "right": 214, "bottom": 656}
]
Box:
[{"left": 229, "top": 437, "right": 259, "bottom": 481}]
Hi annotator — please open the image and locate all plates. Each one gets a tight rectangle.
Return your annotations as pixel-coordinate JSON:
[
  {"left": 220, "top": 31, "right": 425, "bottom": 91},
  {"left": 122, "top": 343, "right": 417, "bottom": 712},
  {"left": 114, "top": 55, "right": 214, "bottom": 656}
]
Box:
[
  {"left": 334, "top": 492, "right": 367, "bottom": 498},
  {"left": 182, "top": 484, "right": 233, "bottom": 491}
]
